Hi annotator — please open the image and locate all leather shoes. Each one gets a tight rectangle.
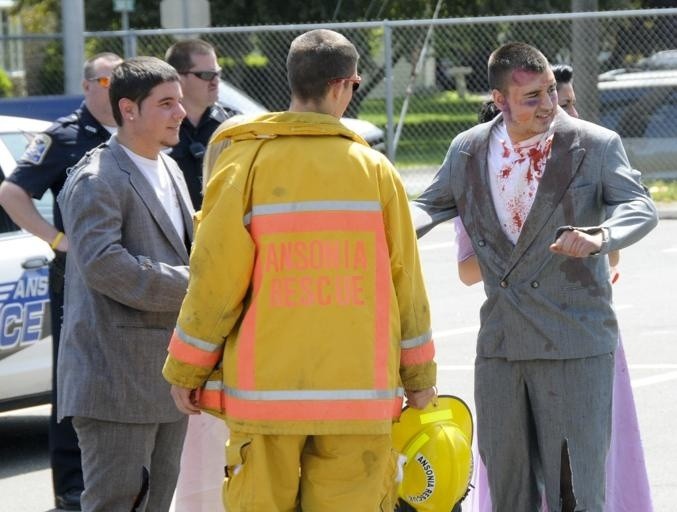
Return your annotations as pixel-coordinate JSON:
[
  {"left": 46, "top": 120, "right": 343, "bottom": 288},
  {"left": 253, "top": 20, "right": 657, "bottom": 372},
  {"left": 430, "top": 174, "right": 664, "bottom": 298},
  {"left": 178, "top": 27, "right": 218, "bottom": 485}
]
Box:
[{"left": 55, "top": 489, "right": 83, "bottom": 510}]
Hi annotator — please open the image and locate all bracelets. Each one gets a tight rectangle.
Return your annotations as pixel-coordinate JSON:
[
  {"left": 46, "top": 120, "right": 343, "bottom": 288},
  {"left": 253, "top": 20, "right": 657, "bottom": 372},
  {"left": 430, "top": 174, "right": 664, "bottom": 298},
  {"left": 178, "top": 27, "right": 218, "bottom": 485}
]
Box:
[{"left": 51, "top": 232, "right": 63, "bottom": 250}]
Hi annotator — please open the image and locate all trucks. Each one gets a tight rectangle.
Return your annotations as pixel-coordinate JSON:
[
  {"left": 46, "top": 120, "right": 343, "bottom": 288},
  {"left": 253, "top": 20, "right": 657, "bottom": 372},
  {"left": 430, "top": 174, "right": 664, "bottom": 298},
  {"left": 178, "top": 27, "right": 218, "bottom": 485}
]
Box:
[{"left": 0, "top": 76, "right": 391, "bottom": 162}]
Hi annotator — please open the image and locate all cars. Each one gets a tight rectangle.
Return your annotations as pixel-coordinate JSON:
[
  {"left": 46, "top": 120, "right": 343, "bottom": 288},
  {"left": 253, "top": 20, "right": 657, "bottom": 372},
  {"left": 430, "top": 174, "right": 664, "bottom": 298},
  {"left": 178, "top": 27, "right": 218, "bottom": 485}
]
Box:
[
  {"left": 598, "top": 49, "right": 677, "bottom": 182},
  {"left": 0, "top": 109, "right": 55, "bottom": 415}
]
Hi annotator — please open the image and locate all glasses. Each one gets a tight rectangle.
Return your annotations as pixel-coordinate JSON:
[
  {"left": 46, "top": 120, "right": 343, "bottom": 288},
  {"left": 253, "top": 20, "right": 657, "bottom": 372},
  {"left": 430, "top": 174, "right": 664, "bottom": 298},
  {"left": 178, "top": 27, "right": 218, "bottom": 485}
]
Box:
[
  {"left": 88, "top": 77, "right": 110, "bottom": 87},
  {"left": 179, "top": 68, "right": 222, "bottom": 80},
  {"left": 328, "top": 76, "right": 361, "bottom": 92}
]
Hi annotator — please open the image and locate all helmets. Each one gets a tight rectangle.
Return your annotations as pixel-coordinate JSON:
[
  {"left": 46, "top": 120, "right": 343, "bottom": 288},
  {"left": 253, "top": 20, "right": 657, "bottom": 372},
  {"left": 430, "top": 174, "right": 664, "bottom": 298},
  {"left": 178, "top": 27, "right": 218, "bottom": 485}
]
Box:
[{"left": 393, "top": 394, "right": 471, "bottom": 512}]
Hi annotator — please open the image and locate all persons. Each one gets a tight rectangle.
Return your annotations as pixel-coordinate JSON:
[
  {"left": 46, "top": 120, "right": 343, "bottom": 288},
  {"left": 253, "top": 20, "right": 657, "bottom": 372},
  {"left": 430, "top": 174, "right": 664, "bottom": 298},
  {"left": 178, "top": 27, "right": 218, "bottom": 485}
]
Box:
[
  {"left": 454, "top": 64, "right": 654, "bottom": 512},
  {"left": 0, "top": 49, "right": 125, "bottom": 512},
  {"left": 164, "top": 40, "right": 245, "bottom": 210},
  {"left": 56, "top": 56, "right": 200, "bottom": 512},
  {"left": 409, "top": 41, "right": 658, "bottom": 512},
  {"left": 162, "top": 29, "right": 438, "bottom": 512}
]
